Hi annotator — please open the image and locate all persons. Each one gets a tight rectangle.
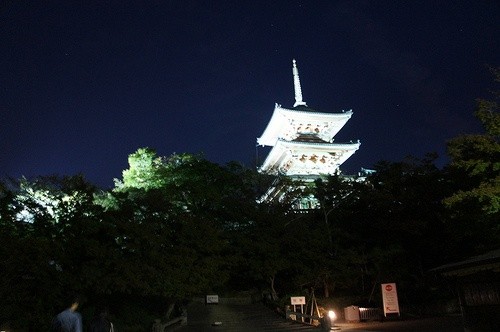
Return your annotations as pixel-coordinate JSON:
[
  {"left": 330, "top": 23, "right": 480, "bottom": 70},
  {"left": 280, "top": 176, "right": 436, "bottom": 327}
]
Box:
[
  {"left": 56, "top": 297, "right": 83, "bottom": 332},
  {"left": 319, "top": 310, "right": 331, "bottom": 332}
]
